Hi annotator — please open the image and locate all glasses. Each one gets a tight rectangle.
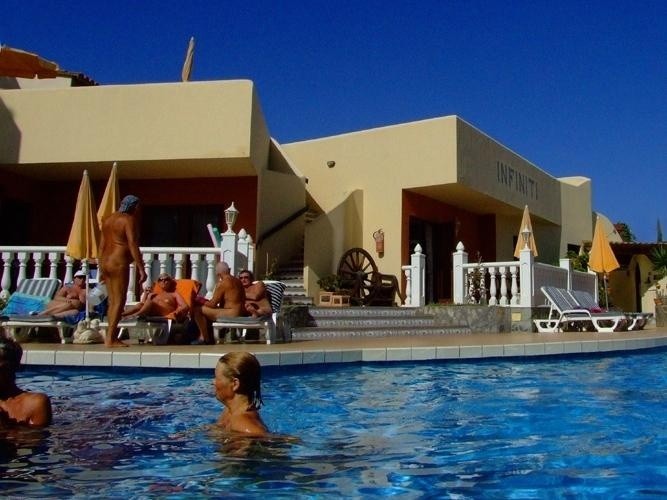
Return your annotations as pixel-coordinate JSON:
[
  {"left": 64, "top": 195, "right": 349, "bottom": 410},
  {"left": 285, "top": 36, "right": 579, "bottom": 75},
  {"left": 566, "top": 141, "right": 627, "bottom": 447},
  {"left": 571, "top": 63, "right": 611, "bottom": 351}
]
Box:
[
  {"left": 158, "top": 277, "right": 169, "bottom": 281},
  {"left": 239, "top": 276, "right": 249, "bottom": 279}
]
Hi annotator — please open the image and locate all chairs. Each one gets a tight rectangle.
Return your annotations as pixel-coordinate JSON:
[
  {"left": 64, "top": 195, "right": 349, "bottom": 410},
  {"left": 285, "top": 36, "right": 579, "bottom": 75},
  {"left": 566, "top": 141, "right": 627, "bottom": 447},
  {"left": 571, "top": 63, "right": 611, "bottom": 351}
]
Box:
[
  {"left": 532, "top": 286, "right": 654, "bottom": 333},
  {"left": 0, "top": 277, "right": 63, "bottom": 341},
  {"left": 1, "top": 279, "right": 105, "bottom": 345},
  {"left": 98, "top": 277, "right": 203, "bottom": 346},
  {"left": 211, "top": 281, "right": 292, "bottom": 345}
]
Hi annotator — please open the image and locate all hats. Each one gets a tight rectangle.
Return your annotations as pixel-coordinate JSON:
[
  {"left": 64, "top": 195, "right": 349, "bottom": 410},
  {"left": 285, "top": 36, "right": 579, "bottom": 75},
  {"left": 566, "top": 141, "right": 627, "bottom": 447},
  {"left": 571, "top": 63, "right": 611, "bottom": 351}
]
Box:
[{"left": 74, "top": 270, "right": 86, "bottom": 277}]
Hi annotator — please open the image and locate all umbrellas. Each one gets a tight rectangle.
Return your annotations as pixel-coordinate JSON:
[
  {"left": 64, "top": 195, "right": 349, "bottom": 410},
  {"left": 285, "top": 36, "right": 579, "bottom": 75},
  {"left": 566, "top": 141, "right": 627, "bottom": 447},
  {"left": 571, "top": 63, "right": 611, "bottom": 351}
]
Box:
[
  {"left": 513, "top": 204, "right": 539, "bottom": 261},
  {"left": 589, "top": 214, "right": 621, "bottom": 312},
  {"left": 65, "top": 169, "right": 101, "bottom": 318},
  {"left": 96, "top": 161, "right": 121, "bottom": 228}
]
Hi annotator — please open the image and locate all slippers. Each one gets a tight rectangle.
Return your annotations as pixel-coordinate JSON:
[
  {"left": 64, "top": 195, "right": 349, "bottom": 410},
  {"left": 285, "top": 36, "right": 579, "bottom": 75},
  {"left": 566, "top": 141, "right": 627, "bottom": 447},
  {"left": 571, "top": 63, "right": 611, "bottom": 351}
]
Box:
[{"left": 190, "top": 338, "right": 209, "bottom": 345}]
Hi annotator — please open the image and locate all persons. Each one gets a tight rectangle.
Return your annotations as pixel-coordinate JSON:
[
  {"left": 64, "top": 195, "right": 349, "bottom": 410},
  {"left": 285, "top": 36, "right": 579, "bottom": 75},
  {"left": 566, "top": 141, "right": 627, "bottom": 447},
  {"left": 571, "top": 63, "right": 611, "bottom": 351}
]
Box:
[
  {"left": 201, "top": 351, "right": 304, "bottom": 478},
  {"left": 36, "top": 269, "right": 88, "bottom": 320},
  {"left": 189, "top": 260, "right": 247, "bottom": 346},
  {"left": 0, "top": 336, "right": 53, "bottom": 429},
  {"left": 238, "top": 269, "right": 272, "bottom": 320},
  {"left": 96, "top": 194, "right": 149, "bottom": 349},
  {"left": 117, "top": 272, "right": 189, "bottom": 322}
]
